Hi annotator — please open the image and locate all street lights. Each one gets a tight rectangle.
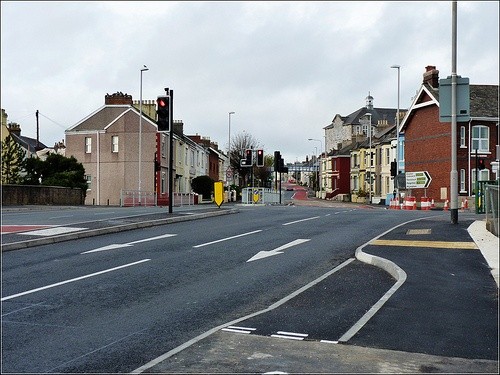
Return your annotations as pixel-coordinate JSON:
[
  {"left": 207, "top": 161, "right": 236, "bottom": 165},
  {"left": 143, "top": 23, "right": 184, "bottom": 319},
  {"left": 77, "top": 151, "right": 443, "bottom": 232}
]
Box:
[
  {"left": 138, "top": 69, "right": 150, "bottom": 205},
  {"left": 228, "top": 111, "right": 235, "bottom": 202},
  {"left": 390, "top": 65, "right": 401, "bottom": 196},
  {"left": 365, "top": 113, "right": 373, "bottom": 204},
  {"left": 308, "top": 138, "right": 322, "bottom": 199}
]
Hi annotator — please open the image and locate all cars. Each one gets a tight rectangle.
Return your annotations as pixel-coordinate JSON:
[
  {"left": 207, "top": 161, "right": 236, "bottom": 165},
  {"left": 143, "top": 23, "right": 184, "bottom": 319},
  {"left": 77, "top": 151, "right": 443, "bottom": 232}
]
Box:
[{"left": 286, "top": 186, "right": 293, "bottom": 191}]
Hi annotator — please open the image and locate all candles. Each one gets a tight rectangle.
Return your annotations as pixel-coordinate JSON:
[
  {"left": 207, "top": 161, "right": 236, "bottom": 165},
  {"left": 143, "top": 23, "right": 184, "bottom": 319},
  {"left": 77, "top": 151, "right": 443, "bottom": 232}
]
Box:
[{"left": 350, "top": 188, "right": 361, "bottom": 203}]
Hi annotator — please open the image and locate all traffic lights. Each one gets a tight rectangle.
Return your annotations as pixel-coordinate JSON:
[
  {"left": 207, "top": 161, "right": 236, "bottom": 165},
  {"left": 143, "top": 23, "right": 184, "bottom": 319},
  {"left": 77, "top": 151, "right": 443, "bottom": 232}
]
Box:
[
  {"left": 156, "top": 96, "right": 171, "bottom": 133},
  {"left": 246, "top": 149, "right": 252, "bottom": 167},
  {"left": 478, "top": 158, "right": 485, "bottom": 170},
  {"left": 256, "top": 150, "right": 264, "bottom": 167}
]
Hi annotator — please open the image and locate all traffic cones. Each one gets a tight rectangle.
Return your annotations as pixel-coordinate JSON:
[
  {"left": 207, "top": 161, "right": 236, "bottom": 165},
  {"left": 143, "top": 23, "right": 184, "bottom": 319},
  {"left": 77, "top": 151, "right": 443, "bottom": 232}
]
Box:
[
  {"left": 426, "top": 198, "right": 430, "bottom": 210},
  {"left": 431, "top": 197, "right": 436, "bottom": 208},
  {"left": 413, "top": 198, "right": 417, "bottom": 210},
  {"left": 464, "top": 199, "right": 469, "bottom": 209},
  {"left": 443, "top": 200, "right": 448, "bottom": 211},
  {"left": 388, "top": 197, "right": 401, "bottom": 210},
  {"left": 461, "top": 199, "right": 465, "bottom": 210}
]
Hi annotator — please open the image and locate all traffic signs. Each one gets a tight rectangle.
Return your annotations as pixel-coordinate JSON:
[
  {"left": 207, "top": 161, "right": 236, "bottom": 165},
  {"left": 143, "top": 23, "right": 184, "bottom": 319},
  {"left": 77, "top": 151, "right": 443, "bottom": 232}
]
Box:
[{"left": 405, "top": 171, "right": 432, "bottom": 189}]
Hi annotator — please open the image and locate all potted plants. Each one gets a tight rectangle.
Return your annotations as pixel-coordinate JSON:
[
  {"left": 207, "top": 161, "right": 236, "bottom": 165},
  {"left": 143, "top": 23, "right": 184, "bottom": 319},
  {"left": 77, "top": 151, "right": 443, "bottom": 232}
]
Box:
[{"left": 356, "top": 189, "right": 369, "bottom": 204}]
